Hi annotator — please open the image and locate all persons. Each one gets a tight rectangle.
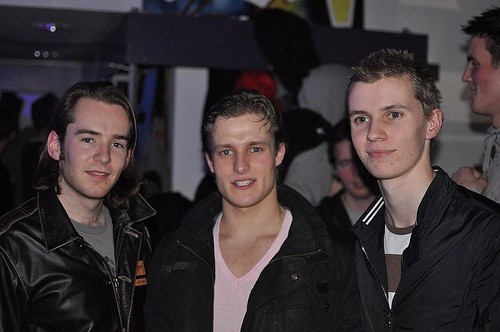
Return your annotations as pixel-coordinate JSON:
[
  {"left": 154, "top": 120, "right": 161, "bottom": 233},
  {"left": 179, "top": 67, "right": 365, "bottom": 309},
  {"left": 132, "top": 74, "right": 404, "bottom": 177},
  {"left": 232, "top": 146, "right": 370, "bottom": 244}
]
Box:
[
  {"left": 140, "top": 86, "right": 343, "bottom": 332},
  {"left": 282, "top": 139, "right": 342, "bottom": 207},
  {"left": 0, "top": 87, "right": 222, "bottom": 230},
  {"left": 326, "top": 49, "right": 500, "bottom": 332},
  {"left": 315, "top": 117, "right": 383, "bottom": 241},
  {"left": 446, "top": 6, "right": 500, "bottom": 203},
  {"left": 0, "top": 78, "right": 175, "bottom": 332}
]
